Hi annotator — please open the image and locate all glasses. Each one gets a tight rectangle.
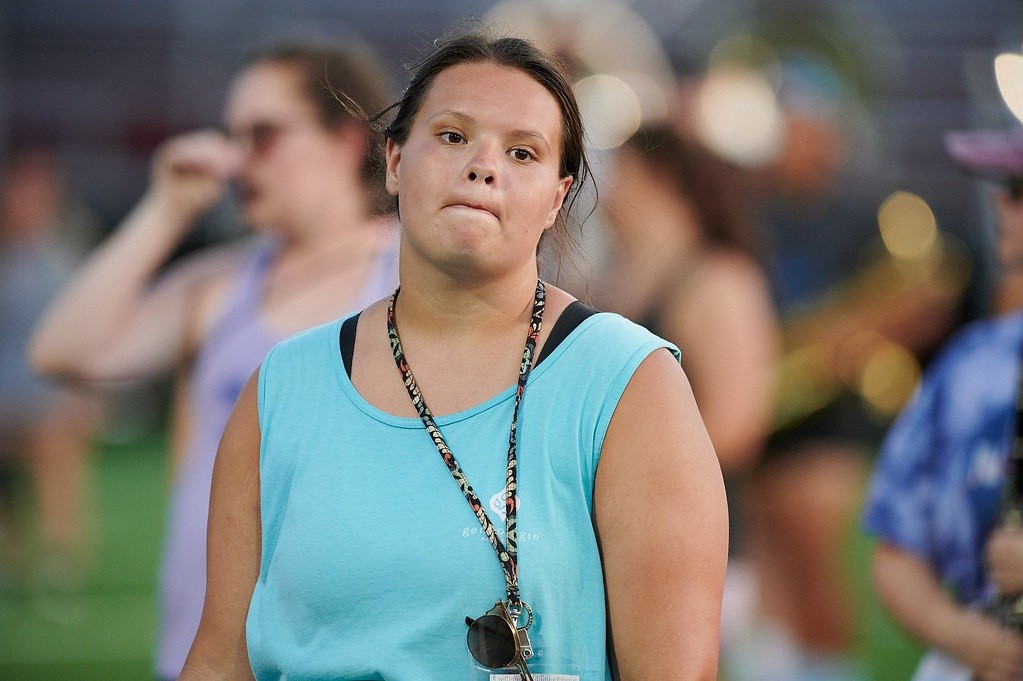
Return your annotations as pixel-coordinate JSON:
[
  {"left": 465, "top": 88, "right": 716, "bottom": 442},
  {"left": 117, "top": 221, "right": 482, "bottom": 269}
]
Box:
[
  {"left": 220, "top": 119, "right": 327, "bottom": 156},
  {"left": 466, "top": 603, "right": 533, "bottom": 681}
]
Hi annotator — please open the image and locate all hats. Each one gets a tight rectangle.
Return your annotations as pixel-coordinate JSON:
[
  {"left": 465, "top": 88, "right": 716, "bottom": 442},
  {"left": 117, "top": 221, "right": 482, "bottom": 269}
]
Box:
[{"left": 943, "top": 127, "right": 1023, "bottom": 177}]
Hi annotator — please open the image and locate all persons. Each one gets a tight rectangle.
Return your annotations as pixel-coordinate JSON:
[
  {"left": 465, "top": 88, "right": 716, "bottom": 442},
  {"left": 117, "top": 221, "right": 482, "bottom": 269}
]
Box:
[
  {"left": 178, "top": 37, "right": 730, "bottom": 681},
  {"left": 28, "top": 50, "right": 782, "bottom": 681},
  {"left": 860, "top": 132, "right": 1023, "bottom": 681}
]
